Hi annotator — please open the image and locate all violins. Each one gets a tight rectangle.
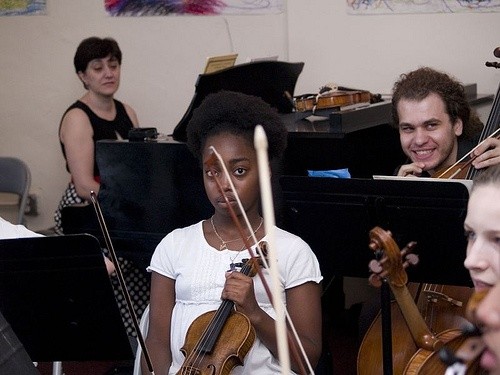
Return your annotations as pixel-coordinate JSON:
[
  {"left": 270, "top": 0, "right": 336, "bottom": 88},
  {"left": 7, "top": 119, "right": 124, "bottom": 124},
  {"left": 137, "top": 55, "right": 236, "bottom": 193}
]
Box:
[
  {"left": 366, "top": 224, "right": 488, "bottom": 375},
  {"left": 174, "top": 241, "right": 268, "bottom": 375},
  {"left": 293, "top": 85, "right": 386, "bottom": 110}
]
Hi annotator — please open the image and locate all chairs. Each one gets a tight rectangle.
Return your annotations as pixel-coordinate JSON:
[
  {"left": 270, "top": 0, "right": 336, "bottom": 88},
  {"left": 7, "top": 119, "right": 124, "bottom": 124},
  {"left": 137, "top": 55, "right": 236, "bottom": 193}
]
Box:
[{"left": 0, "top": 157, "right": 31, "bottom": 225}]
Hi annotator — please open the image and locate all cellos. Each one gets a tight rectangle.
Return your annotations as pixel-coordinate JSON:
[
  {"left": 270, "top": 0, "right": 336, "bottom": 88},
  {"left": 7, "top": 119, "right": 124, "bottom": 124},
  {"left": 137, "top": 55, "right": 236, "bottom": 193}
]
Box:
[{"left": 355, "top": 45, "right": 500, "bottom": 375}]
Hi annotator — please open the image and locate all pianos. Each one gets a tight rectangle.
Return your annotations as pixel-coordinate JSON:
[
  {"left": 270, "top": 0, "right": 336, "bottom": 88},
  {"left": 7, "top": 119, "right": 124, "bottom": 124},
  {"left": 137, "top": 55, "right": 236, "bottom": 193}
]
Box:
[{"left": 60, "top": 86, "right": 493, "bottom": 327}]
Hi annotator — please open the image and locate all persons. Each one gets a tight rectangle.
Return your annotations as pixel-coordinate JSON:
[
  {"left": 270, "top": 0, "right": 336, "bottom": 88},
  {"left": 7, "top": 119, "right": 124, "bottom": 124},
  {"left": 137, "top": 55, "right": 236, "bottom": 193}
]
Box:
[
  {"left": 392, "top": 67, "right": 500, "bottom": 179},
  {"left": 140, "top": 91, "right": 325, "bottom": 375},
  {"left": 476, "top": 283, "right": 500, "bottom": 375},
  {"left": 54, "top": 36, "right": 142, "bottom": 236},
  {"left": 0, "top": 217, "right": 115, "bottom": 275},
  {"left": 461, "top": 163, "right": 499, "bottom": 302}
]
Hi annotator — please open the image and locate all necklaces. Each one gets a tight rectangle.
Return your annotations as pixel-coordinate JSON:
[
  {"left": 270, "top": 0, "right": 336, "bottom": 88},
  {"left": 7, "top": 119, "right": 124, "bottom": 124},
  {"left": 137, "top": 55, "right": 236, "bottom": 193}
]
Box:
[{"left": 211, "top": 215, "right": 264, "bottom": 270}]
